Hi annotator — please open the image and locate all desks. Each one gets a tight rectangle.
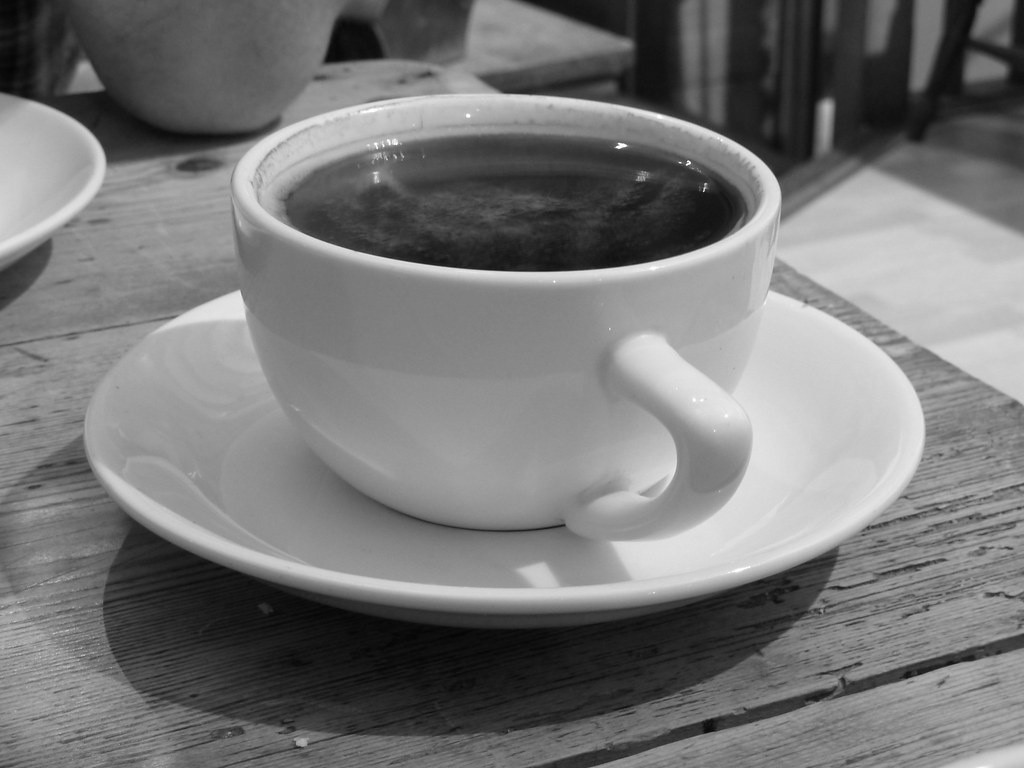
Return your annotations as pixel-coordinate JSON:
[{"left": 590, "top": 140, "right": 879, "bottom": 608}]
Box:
[{"left": 0, "top": 57, "right": 1024, "bottom": 767}]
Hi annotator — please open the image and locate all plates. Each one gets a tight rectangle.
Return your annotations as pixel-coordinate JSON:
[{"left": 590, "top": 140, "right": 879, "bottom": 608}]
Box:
[
  {"left": 80, "top": 286, "right": 928, "bottom": 629},
  {"left": 0, "top": 91, "right": 107, "bottom": 272}
]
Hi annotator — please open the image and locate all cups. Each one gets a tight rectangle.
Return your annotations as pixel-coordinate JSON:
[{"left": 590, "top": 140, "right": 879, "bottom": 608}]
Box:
[
  {"left": 63, "top": 0, "right": 342, "bottom": 137},
  {"left": 229, "top": 91, "right": 783, "bottom": 542}
]
